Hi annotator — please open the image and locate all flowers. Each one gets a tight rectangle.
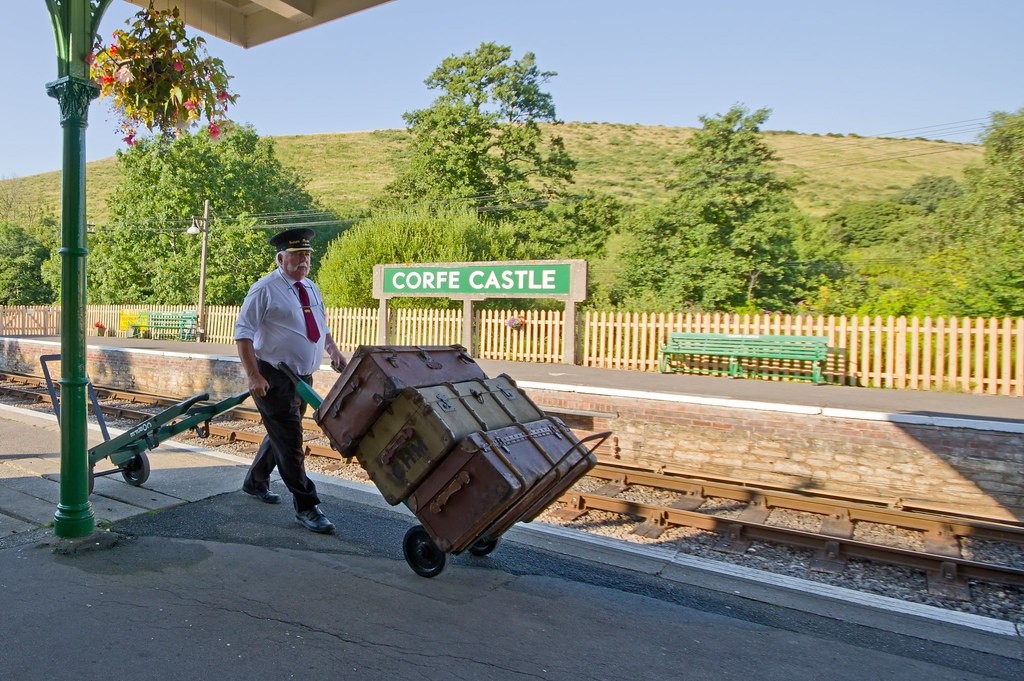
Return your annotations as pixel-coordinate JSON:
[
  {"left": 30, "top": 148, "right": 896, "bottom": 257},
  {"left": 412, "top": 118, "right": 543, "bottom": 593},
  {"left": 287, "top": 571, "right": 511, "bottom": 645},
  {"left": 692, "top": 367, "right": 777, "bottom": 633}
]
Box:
[
  {"left": 85, "top": 0, "right": 240, "bottom": 147},
  {"left": 94, "top": 322, "right": 105, "bottom": 329},
  {"left": 507, "top": 314, "right": 527, "bottom": 330}
]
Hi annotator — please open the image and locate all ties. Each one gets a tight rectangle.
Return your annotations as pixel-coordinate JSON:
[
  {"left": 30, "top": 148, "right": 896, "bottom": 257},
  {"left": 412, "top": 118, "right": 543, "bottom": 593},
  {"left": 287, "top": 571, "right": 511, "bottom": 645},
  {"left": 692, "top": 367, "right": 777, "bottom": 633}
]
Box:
[{"left": 293, "top": 282, "right": 321, "bottom": 343}]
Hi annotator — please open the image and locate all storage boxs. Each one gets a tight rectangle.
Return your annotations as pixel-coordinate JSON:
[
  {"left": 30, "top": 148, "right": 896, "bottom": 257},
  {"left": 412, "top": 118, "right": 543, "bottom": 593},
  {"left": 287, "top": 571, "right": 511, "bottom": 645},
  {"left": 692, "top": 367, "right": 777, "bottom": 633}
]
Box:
[
  {"left": 408, "top": 417, "right": 598, "bottom": 552},
  {"left": 312, "top": 343, "right": 490, "bottom": 458},
  {"left": 355, "top": 375, "right": 547, "bottom": 506}
]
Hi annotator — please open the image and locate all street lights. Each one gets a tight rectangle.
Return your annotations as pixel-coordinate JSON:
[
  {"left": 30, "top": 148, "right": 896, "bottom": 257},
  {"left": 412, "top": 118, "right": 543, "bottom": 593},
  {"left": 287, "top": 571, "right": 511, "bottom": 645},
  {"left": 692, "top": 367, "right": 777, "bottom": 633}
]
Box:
[{"left": 187, "top": 200, "right": 212, "bottom": 342}]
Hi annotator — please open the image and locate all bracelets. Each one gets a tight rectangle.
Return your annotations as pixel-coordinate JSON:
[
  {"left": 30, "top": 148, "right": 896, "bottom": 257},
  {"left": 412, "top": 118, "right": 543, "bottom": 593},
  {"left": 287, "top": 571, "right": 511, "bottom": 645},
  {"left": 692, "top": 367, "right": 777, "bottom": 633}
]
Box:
[{"left": 325, "top": 341, "right": 335, "bottom": 352}]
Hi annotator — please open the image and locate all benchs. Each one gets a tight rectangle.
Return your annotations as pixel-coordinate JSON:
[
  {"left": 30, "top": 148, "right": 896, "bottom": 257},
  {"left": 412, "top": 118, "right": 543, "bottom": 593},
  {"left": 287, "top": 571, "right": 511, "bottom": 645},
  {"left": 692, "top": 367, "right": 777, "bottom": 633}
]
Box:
[
  {"left": 657, "top": 332, "right": 829, "bottom": 385},
  {"left": 130, "top": 311, "right": 198, "bottom": 341}
]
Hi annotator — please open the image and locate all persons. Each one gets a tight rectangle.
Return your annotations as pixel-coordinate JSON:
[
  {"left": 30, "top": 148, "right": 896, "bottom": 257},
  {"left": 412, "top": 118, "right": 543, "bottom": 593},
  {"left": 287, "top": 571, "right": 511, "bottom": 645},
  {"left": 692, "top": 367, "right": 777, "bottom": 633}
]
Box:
[{"left": 234, "top": 228, "right": 348, "bottom": 532}]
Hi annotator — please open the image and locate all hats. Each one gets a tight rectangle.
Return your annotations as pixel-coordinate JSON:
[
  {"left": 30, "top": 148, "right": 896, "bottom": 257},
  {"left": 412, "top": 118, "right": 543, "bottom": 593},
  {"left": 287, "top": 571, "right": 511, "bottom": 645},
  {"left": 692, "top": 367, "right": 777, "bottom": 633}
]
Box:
[{"left": 270, "top": 229, "right": 315, "bottom": 252}]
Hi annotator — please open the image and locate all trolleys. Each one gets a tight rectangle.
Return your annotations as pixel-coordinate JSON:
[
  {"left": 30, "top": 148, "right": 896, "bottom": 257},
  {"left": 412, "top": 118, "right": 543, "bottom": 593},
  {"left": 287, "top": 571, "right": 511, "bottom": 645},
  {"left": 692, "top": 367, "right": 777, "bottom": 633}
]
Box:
[
  {"left": 39, "top": 353, "right": 270, "bottom": 497},
  {"left": 277, "top": 356, "right": 612, "bottom": 578}
]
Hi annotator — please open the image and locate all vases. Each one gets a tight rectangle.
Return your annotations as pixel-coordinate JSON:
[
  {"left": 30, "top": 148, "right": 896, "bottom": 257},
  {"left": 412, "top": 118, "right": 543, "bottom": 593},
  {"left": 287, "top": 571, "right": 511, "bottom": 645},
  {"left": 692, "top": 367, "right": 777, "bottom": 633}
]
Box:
[{"left": 94, "top": 327, "right": 104, "bottom": 336}]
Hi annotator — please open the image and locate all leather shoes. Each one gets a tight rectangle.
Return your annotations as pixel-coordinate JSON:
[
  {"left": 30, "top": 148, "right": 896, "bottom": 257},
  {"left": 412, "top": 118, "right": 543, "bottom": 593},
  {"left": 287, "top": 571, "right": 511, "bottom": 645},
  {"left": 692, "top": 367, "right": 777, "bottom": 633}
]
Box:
[
  {"left": 242, "top": 484, "right": 282, "bottom": 504},
  {"left": 295, "top": 504, "right": 336, "bottom": 534}
]
